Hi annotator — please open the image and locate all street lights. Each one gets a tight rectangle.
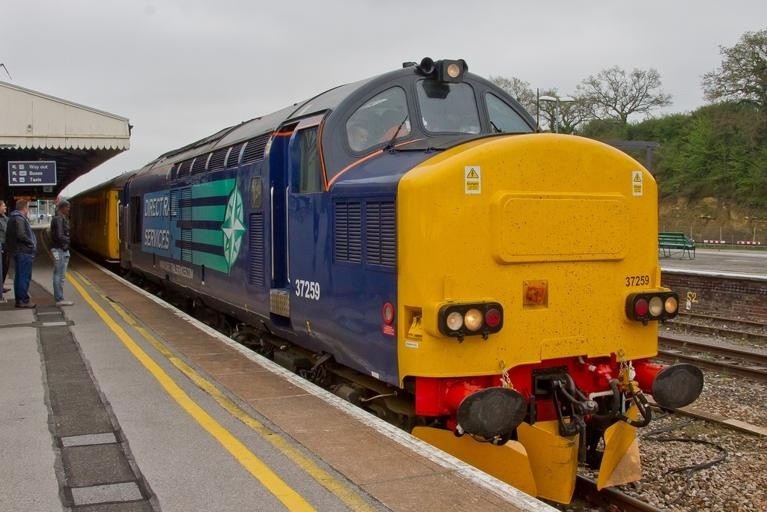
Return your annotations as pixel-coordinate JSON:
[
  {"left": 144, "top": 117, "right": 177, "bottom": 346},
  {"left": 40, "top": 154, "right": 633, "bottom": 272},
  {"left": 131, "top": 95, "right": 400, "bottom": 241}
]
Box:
[{"left": 539, "top": 96, "right": 575, "bottom": 134}]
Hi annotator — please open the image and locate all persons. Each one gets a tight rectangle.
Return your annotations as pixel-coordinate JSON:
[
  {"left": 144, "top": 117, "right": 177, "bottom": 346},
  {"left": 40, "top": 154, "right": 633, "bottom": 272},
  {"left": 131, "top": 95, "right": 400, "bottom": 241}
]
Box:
[
  {"left": 48, "top": 200, "right": 74, "bottom": 306},
  {"left": 5, "top": 198, "right": 37, "bottom": 309},
  {"left": 348, "top": 124, "right": 370, "bottom": 150},
  {"left": 0, "top": 199, "right": 11, "bottom": 292}
]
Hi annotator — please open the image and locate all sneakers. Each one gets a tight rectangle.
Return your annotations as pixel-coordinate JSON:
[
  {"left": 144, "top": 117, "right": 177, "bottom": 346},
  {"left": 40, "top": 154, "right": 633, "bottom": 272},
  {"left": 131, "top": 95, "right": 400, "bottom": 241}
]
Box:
[
  {"left": 55, "top": 300, "right": 73, "bottom": 306},
  {"left": 2, "top": 287, "right": 11, "bottom": 293},
  {"left": 15, "top": 300, "right": 36, "bottom": 308}
]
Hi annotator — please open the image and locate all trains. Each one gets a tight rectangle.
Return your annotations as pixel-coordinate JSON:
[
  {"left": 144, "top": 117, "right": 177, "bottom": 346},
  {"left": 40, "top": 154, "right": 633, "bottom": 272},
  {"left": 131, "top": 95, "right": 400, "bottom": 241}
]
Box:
[{"left": 68, "top": 57, "right": 703, "bottom": 504}]
